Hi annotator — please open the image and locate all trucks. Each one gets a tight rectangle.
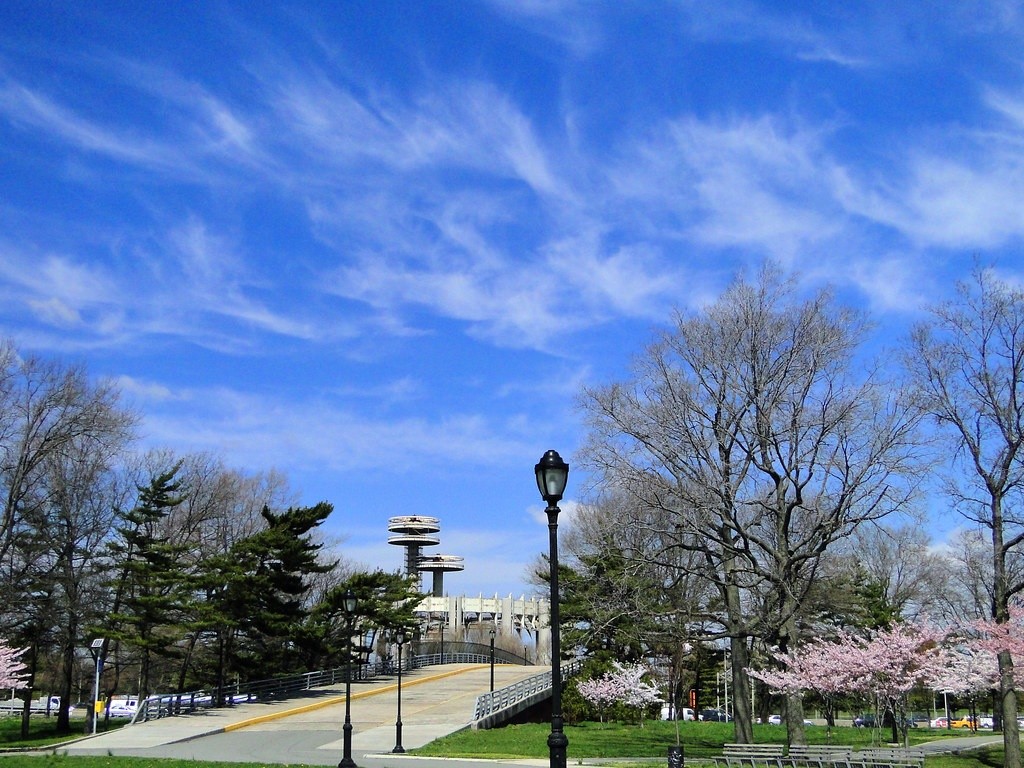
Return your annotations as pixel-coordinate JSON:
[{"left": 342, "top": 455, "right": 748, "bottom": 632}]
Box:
[{"left": 0, "top": 696, "right": 74, "bottom": 716}]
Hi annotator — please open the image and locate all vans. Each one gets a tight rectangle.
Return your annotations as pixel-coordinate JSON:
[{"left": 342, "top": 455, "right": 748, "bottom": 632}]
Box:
[
  {"left": 640, "top": 701, "right": 674, "bottom": 720},
  {"left": 656, "top": 708, "right": 704, "bottom": 722},
  {"left": 103, "top": 700, "right": 138, "bottom": 715}
]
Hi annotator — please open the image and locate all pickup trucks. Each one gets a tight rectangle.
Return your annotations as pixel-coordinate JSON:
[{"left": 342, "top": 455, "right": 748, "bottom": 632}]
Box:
[{"left": 949, "top": 717, "right": 979, "bottom": 728}]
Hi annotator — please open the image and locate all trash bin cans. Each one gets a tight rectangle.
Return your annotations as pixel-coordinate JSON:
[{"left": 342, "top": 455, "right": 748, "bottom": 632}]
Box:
[{"left": 668, "top": 746, "right": 685, "bottom": 768}]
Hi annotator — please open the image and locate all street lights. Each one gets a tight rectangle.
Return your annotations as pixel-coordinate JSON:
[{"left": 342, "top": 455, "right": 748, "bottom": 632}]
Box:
[
  {"left": 524, "top": 644, "right": 527, "bottom": 666},
  {"left": 440, "top": 621, "right": 446, "bottom": 664},
  {"left": 489, "top": 630, "right": 496, "bottom": 692},
  {"left": 534, "top": 449, "right": 570, "bottom": 768},
  {"left": 333, "top": 584, "right": 358, "bottom": 768},
  {"left": 392, "top": 624, "right": 406, "bottom": 753}
]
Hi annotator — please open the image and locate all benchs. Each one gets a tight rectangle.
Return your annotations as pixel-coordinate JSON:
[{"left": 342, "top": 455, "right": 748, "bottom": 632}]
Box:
[{"left": 710, "top": 743, "right": 925, "bottom": 768}]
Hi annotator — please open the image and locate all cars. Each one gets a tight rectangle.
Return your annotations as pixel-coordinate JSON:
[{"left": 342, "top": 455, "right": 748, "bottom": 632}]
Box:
[
  {"left": 103, "top": 705, "right": 137, "bottom": 718},
  {"left": 700, "top": 709, "right": 731, "bottom": 722},
  {"left": 896, "top": 715, "right": 918, "bottom": 728},
  {"left": 804, "top": 720, "right": 814, "bottom": 726},
  {"left": 851, "top": 714, "right": 883, "bottom": 728},
  {"left": 927, "top": 717, "right": 952, "bottom": 728},
  {"left": 756, "top": 715, "right": 780, "bottom": 725},
  {"left": 1016, "top": 717, "right": 1024, "bottom": 726},
  {"left": 912, "top": 715, "right": 931, "bottom": 722},
  {"left": 976, "top": 715, "right": 994, "bottom": 727}
]
